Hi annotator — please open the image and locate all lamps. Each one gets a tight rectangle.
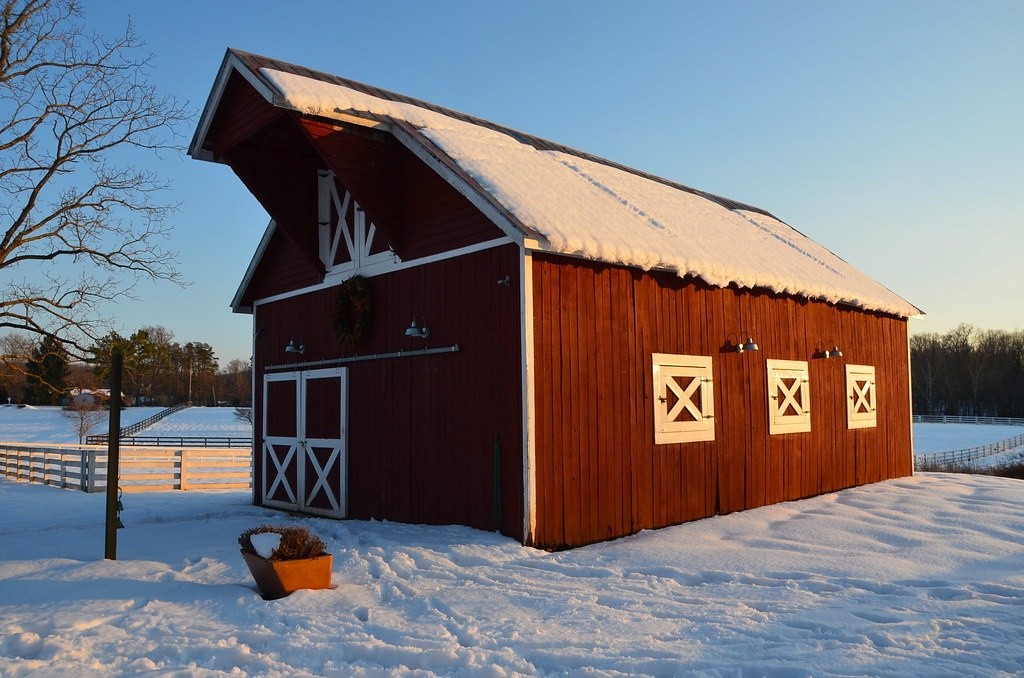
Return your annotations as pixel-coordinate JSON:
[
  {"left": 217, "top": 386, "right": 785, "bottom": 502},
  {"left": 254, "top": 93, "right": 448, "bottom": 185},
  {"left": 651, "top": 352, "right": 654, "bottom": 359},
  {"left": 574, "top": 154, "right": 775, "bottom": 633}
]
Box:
[
  {"left": 495, "top": 275, "right": 513, "bottom": 287},
  {"left": 405, "top": 312, "right": 432, "bottom": 339},
  {"left": 735, "top": 331, "right": 759, "bottom": 355},
  {"left": 286, "top": 333, "right": 307, "bottom": 355},
  {"left": 824, "top": 341, "right": 843, "bottom": 359}
]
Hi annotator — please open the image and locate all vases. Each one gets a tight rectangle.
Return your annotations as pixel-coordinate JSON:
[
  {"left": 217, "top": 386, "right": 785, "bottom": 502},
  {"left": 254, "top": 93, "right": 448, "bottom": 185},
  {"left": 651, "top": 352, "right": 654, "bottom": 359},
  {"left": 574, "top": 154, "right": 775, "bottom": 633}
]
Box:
[{"left": 239, "top": 547, "right": 335, "bottom": 600}]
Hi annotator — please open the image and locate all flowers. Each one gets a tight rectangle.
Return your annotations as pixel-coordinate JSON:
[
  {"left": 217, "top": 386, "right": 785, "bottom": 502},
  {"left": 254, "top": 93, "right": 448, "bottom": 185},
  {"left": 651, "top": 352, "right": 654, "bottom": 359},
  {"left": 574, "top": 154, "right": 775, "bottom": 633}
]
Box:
[{"left": 236, "top": 522, "right": 329, "bottom": 563}]
